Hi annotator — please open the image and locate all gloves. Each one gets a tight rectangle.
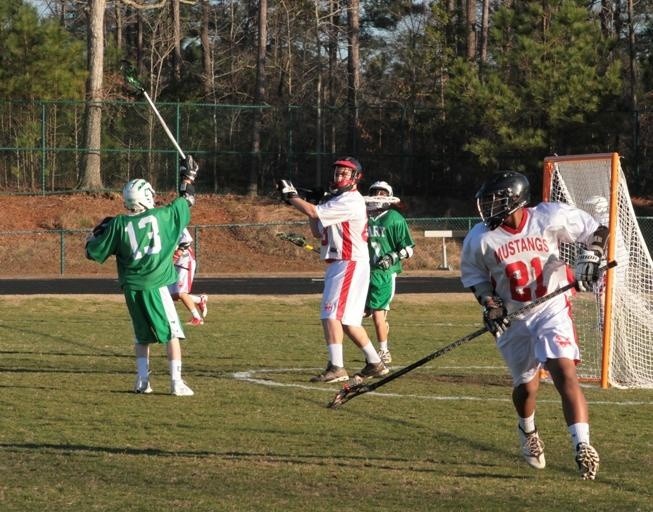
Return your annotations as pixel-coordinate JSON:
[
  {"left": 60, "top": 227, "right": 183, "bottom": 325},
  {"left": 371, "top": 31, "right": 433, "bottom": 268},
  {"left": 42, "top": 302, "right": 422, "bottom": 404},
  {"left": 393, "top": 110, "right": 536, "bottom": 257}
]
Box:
[
  {"left": 275, "top": 179, "right": 298, "bottom": 205},
  {"left": 377, "top": 253, "right": 394, "bottom": 270},
  {"left": 180, "top": 155, "right": 198, "bottom": 182}
]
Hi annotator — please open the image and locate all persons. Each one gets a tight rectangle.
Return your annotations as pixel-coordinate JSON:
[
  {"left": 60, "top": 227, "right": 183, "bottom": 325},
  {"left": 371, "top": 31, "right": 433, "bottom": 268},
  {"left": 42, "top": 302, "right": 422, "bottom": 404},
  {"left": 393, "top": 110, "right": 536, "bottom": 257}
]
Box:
[
  {"left": 582, "top": 186, "right": 609, "bottom": 230},
  {"left": 84, "top": 153, "right": 200, "bottom": 396},
  {"left": 362, "top": 181, "right": 415, "bottom": 365},
  {"left": 274, "top": 155, "right": 389, "bottom": 384},
  {"left": 166, "top": 227, "right": 208, "bottom": 327},
  {"left": 461, "top": 170, "right": 608, "bottom": 479}
]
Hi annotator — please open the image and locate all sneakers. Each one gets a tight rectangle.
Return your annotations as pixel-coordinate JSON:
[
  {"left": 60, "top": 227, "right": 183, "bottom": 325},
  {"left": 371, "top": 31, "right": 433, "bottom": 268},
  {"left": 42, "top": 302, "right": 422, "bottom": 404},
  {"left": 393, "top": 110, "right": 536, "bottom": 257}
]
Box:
[
  {"left": 310, "top": 360, "right": 349, "bottom": 383},
  {"left": 198, "top": 295, "right": 208, "bottom": 317},
  {"left": 170, "top": 379, "right": 194, "bottom": 396},
  {"left": 185, "top": 316, "right": 204, "bottom": 326},
  {"left": 134, "top": 377, "right": 153, "bottom": 394},
  {"left": 575, "top": 442, "right": 599, "bottom": 480},
  {"left": 576, "top": 247, "right": 602, "bottom": 293},
  {"left": 518, "top": 424, "right": 546, "bottom": 469},
  {"left": 353, "top": 358, "right": 389, "bottom": 379},
  {"left": 483, "top": 296, "right": 511, "bottom": 338},
  {"left": 377, "top": 350, "right": 392, "bottom": 364}
]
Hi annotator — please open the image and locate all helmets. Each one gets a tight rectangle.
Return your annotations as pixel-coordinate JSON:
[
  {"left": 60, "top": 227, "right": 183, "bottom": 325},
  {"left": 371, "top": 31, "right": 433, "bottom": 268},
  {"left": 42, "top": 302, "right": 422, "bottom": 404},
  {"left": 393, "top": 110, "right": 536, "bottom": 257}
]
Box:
[
  {"left": 368, "top": 181, "right": 393, "bottom": 210},
  {"left": 476, "top": 170, "right": 530, "bottom": 231},
  {"left": 329, "top": 156, "right": 362, "bottom": 196},
  {"left": 122, "top": 179, "right": 155, "bottom": 211},
  {"left": 584, "top": 196, "right": 609, "bottom": 214}
]
